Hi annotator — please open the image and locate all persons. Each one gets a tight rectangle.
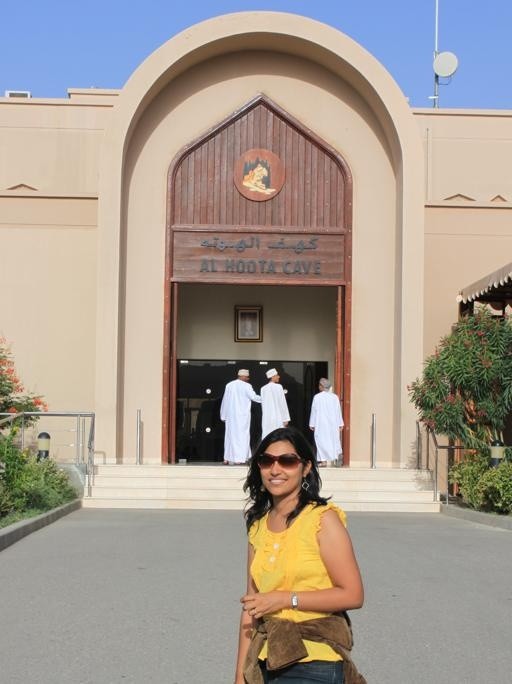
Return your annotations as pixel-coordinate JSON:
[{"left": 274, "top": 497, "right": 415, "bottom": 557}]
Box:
[
  {"left": 219, "top": 367, "right": 262, "bottom": 464},
  {"left": 309, "top": 376, "right": 345, "bottom": 468},
  {"left": 259, "top": 367, "right": 293, "bottom": 443},
  {"left": 233, "top": 424, "right": 367, "bottom": 684}
]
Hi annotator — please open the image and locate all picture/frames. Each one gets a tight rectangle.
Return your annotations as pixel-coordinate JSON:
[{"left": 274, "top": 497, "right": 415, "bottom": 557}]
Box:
[{"left": 234, "top": 302, "right": 264, "bottom": 342}]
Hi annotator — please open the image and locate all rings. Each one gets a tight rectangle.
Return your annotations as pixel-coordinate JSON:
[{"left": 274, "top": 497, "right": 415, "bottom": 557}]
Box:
[{"left": 254, "top": 608, "right": 258, "bottom": 614}]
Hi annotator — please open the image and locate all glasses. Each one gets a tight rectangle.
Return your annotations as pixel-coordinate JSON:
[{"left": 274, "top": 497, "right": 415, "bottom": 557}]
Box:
[{"left": 257, "top": 452, "right": 300, "bottom": 470}]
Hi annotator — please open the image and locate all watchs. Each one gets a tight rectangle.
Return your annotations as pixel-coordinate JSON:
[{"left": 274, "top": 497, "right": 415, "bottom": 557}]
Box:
[{"left": 291, "top": 592, "right": 298, "bottom": 609}]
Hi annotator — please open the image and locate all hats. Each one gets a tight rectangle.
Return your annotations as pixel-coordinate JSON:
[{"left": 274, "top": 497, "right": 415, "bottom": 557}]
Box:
[
  {"left": 237, "top": 369, "right": 250, "bottom": 377},
  {"left": 265, "top": 367, "right": 278, "bottom": 378},
  {"left": 319, "top": 378, "right": 332, "bottom": 388}
]
